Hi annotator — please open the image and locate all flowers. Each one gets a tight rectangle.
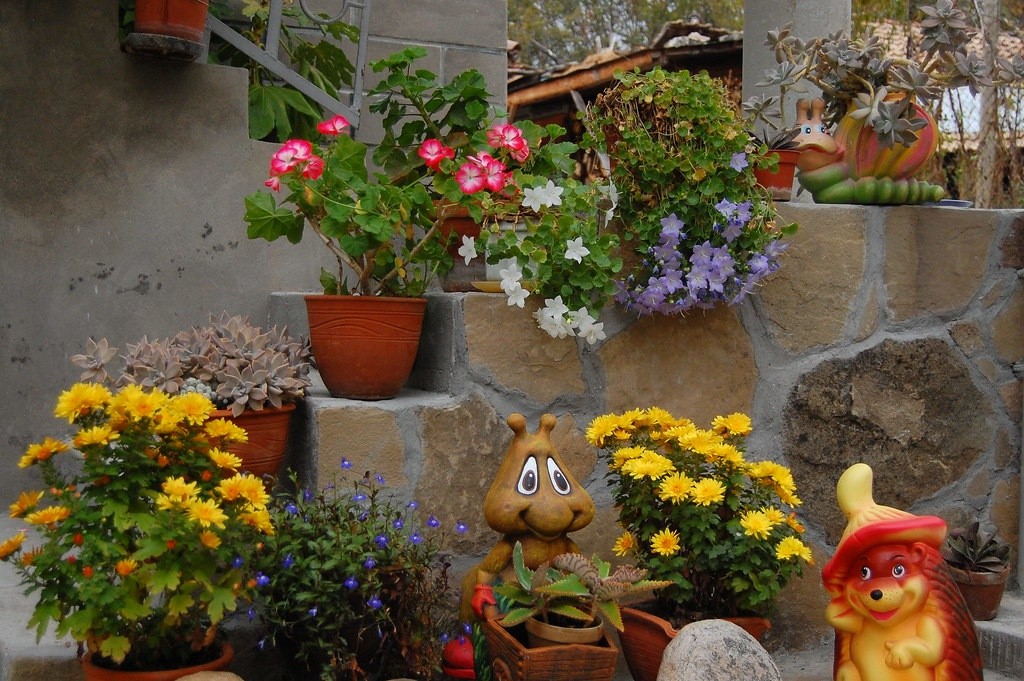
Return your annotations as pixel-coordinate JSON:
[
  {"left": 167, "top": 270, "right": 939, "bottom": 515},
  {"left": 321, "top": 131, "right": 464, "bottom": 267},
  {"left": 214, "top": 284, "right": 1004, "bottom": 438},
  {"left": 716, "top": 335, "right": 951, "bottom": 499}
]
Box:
[
  {"left": 576, "top": 67, "right": 796, "bottom": 316},
  {"left": 244, "top": 46, "right": 530, "bottom": 295},
  {"left": 585, "top": 406, "right": 816, "bottom": 614},
  {"left": 233, "top": 457, "right": 465, "bottom": 651},
  {"left": 0, "top": 380, "right": 275, "bottom": 666},
  {"left": 434, "top": 120, "right": 622, "bottom": 344}
]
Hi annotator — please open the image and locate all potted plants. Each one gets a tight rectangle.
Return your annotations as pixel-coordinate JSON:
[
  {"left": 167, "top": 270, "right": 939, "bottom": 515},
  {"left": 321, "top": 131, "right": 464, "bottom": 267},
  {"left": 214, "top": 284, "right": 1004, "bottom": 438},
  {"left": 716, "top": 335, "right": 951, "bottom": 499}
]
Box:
[
  {"left": 70, "top": 309, "right": 317, "bottom": 508},
  {"left": 492, "top": 541, "right": 671, "bottom": 649},
  {"left": 745, "top": 123, "right": 802, "bottom": 200},
  {"left": 740, "top": 0, "right": 1024, "bottom": 150},
  {"left": 942, "top": 521, "right": 1011, "bottom": 621}
]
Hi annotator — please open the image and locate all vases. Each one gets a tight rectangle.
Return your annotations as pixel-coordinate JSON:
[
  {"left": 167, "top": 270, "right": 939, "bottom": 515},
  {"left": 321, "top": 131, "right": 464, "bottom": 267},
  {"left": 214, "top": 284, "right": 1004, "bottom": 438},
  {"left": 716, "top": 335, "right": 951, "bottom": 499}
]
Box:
[
  {"left": 82, "top": 627, "right": 235, "bottom": 681},
  {"left": 135, "top": 0, "right": 210, "bottom": 43},
  {"left": 423, "top": 198, "right": 486, "bottom": 260},
  {"left": 470, "top": 217, "right": 548, "bottom": 292},
  {"left": 304, "top": 295, "right": 428, "bottom": 401},
  {"left": 617, "top": 598, "right": 772, "bottom": 681}
]
